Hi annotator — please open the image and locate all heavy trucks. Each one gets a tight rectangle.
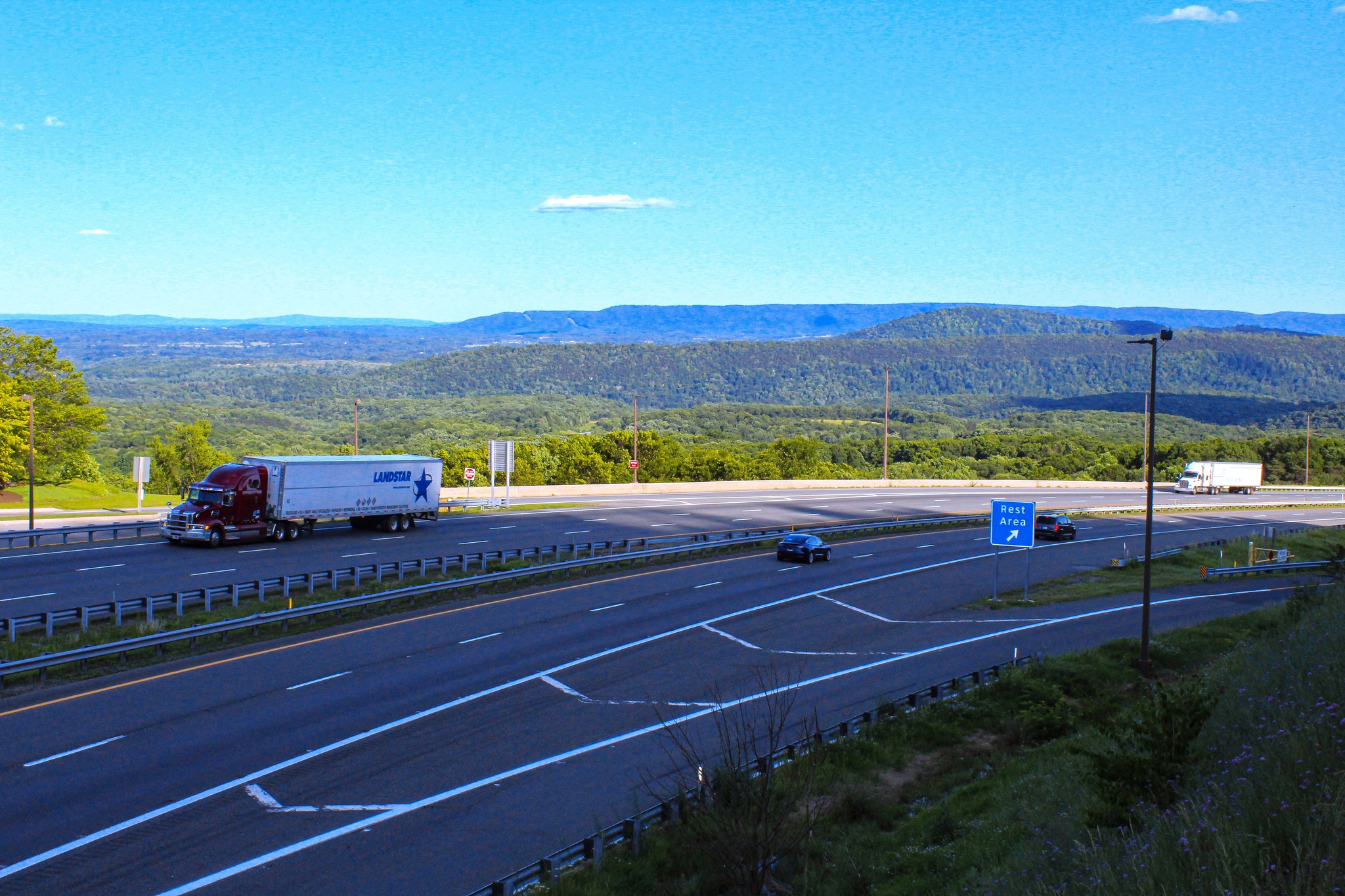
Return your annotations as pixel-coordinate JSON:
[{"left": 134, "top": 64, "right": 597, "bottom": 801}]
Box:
[
  {"left": 159, "top": 455, "right": 444, "bottom": 551},
  {"left": 1174, "top": 461, "right": 1263, "bottom": 496}
]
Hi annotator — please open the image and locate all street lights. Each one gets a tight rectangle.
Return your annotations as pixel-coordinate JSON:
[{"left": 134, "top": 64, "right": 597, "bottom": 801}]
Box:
[
  {"left": 1120, "top": 327, "right": 1177, "bottom": 664},
  {"left": 883, "top": 363, "right": 894, "bottom": 480},
  {"left": 21, "top": 393, "right": 35, "bottom": 548},
  {"left": 1305, "top": 411, "right": 1315, "bottom": 487},
  {"left": 353, "top": 399, "right": 362, "bottom": 454},
  {"left": 631, "top": 395, "right": 646, "bottom": 484}
]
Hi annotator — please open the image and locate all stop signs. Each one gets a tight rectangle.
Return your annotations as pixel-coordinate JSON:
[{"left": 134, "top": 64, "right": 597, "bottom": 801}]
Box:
[{"left": 465, "top": 469, "right": 475, "bottom": 479}]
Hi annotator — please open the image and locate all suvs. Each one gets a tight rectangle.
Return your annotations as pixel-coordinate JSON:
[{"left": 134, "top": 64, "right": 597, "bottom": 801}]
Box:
[{"left": 1035, "top": 515, "right": 1076, "bottom": 542}]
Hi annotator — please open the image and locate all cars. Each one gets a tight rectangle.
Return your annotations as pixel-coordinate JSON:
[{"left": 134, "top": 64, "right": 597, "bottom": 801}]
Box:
[{"left": 776, "top": 533, "right": 832, "bottom": 565}]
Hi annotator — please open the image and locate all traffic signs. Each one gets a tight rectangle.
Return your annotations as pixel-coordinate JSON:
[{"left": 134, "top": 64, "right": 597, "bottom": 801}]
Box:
[{"left": 988, "top": 500, "right": 1036, "bottom": 547}]
